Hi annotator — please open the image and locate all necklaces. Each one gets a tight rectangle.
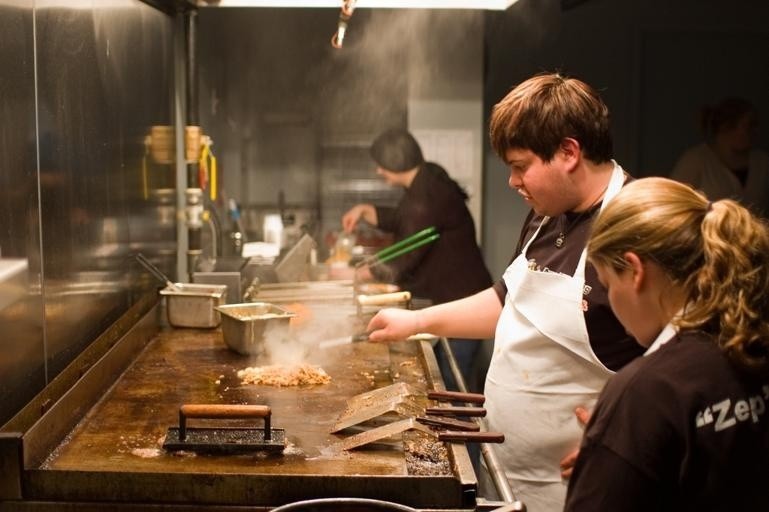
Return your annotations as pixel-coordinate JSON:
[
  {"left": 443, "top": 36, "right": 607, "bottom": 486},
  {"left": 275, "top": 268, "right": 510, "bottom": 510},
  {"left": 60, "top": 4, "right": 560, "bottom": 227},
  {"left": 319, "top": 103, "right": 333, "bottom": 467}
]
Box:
[{"left": 552, "top": 184, "right": 607, "bottom": 248}]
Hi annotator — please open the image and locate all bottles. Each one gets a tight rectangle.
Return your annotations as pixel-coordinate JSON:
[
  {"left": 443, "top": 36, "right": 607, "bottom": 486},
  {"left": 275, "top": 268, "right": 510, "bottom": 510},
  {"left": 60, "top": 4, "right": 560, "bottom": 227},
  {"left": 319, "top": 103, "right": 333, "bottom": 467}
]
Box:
[{"left": 185, "top": 188, "right": 203, "bottom": 251}]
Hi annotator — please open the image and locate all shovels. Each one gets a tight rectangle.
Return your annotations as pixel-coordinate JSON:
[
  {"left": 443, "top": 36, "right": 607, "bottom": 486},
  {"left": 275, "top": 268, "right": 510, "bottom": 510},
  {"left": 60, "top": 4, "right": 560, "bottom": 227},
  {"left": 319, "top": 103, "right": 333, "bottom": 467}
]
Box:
[{"left": 329, "top": 382, "right": 505, "bottom": 451}]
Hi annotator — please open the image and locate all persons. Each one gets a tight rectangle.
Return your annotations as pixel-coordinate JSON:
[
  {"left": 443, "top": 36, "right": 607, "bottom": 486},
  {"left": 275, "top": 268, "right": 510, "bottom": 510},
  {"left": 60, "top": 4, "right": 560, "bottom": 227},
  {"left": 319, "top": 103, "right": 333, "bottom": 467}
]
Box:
[
  {"left": 669, "top": 96, "right": 769, "bottom": 222},
  {"left": 364, "top": 70, "right": 671, "bottom": 511},
  {"left": 340, "top": 128, "right": 497, "bottom": 391},
  {"left": 560, "top": 174, "right": 767, "bottom": 511}
]
